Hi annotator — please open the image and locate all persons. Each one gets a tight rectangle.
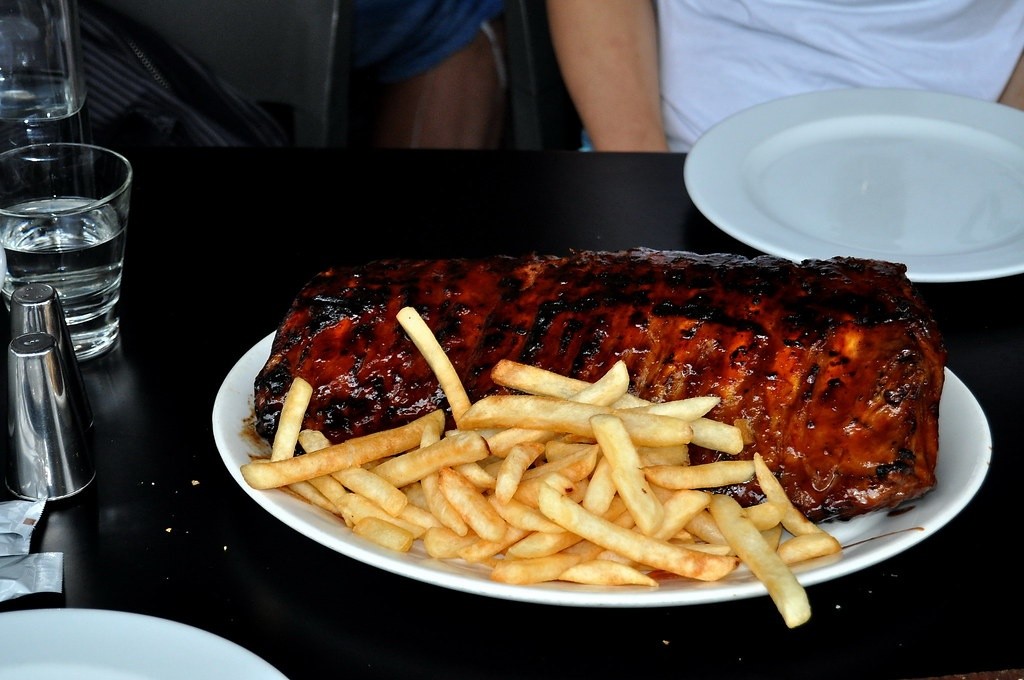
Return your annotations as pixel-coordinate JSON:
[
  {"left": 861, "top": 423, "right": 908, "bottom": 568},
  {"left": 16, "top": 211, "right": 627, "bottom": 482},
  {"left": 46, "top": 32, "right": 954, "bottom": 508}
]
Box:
[
  {"left": 1, "top": 1, "right": 513, "bottom": 149},
  {"left": 545, "top": 0, "right": 1024, "bottom": 154}
]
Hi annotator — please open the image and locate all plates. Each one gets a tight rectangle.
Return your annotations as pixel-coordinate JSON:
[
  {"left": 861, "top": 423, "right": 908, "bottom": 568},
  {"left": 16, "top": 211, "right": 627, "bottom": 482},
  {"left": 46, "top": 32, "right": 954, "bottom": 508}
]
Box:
[
  {"left": 683, "top": 86, "right": 1024, "bottom": 282},
  {"left": 212, "top": 326, "right": 993, "bottom": 605},
  {"left": 0, "top": 608, "right": 287, "bottom": 679}
]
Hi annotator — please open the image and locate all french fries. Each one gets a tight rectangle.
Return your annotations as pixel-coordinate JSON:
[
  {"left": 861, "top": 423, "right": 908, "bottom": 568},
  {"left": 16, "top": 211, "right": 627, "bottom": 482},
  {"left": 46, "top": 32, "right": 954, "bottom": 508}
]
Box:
[{"left": 240, "top": 309, "right": 844, "bottom": 631}]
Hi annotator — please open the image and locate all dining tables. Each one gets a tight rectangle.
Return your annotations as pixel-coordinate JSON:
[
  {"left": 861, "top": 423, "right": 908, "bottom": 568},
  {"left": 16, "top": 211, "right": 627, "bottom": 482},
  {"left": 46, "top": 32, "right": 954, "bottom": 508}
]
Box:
[{"left": 0, "top": 143, "right": 1024, "bottom": 680}]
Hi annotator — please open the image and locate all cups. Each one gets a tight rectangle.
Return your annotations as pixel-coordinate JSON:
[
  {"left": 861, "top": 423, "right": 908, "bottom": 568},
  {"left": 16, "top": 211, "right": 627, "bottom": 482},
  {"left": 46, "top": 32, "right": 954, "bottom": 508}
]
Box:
[
  {"left": 0, "top": 1, "right": 87, "bottom": 121},
  {"left": 0, "top": 142, "right": 133, "bottom": 362}
]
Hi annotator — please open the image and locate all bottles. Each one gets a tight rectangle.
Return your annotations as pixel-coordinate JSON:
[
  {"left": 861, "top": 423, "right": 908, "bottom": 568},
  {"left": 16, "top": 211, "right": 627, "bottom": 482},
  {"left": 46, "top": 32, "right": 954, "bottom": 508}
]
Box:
[{"left": 2, "top": 283, "right": 98, "bottom": 500}]
